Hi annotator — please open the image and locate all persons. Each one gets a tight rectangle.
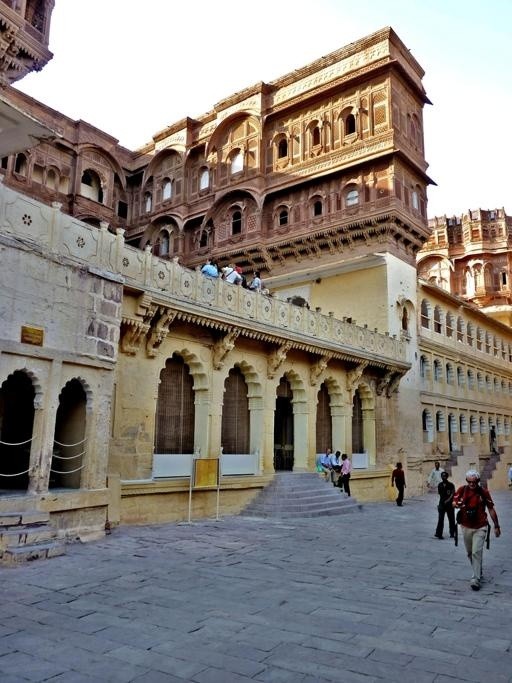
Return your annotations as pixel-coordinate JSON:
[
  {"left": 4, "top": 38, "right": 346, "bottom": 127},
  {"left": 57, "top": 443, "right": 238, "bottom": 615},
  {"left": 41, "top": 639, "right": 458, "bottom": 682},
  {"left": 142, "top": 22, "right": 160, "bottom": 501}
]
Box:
[
  {"left": 391, "top": 462, "right": 406, "bottom": 506},
  {"left": 200, "top": 256, "right": 269, "bottom": 297},
  {"left": 329, "top": 450, "right": 342, "bottom": 473},
  {"left": 432, "top": 472, "right": 456, "bottom": 539},
  {"left": 428, "top": 461, "right": 445, "bottom": 509},
  {"left": 452, "top": 469, "right": 500, "bottom": 591},
  {"left": 336, "top": 454, "right": 352, "bottom": 496},
  {"left": 507, "top": 467, "right": 512, "bottom": 492},
  {"left": 316, "top": 449, "right": 336, "bottom": 487}
]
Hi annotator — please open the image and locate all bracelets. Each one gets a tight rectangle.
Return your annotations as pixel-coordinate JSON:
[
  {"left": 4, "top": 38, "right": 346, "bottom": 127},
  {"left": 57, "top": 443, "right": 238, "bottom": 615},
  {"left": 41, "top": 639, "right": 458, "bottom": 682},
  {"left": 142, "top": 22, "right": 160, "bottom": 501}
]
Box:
[{"left": 494, "top": 526, "right": 500, "bottom": 528}]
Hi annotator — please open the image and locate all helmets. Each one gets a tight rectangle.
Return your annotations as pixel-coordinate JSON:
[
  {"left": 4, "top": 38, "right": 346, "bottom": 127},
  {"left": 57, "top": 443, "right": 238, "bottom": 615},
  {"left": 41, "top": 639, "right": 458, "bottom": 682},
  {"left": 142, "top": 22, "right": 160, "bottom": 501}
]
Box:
[{"left": 466, "top": 470, "right": 480, "bottom": 482}]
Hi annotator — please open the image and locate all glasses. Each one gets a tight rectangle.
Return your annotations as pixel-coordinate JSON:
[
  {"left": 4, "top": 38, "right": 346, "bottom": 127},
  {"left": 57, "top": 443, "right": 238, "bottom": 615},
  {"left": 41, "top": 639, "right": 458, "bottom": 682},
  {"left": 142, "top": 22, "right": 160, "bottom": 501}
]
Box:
[{"left": 466, "top": 478, "right": 478, "bottom": 484}]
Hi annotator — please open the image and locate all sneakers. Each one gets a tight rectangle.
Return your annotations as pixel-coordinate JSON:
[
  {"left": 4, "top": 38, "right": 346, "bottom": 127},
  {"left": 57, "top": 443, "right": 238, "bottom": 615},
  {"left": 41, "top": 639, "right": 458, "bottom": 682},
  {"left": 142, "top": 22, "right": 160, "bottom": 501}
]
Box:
[{"left": 470, "top": 582, "right": 480, "bottom": 591}]
